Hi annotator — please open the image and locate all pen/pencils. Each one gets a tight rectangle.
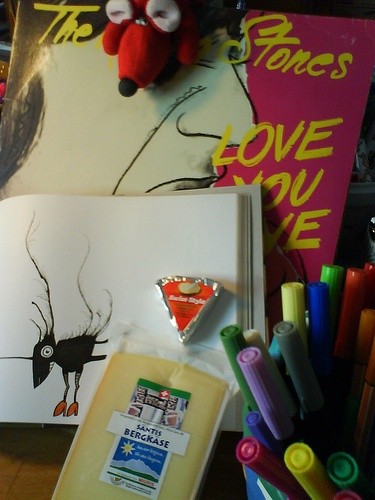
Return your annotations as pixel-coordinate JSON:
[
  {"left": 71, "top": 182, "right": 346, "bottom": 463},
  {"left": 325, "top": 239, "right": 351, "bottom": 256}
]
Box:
[{"left": 218, "top": 262, "right": 375, "bottom": 500}]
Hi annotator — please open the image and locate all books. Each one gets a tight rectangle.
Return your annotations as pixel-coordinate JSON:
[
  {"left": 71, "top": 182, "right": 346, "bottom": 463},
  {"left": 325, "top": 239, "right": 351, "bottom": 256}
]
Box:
[
  {"left": 0, "top": 184, "right": 267, "bottom": 432},
  {"left": 1, "top": 0, "right": 375, "bottom": 348}
]
